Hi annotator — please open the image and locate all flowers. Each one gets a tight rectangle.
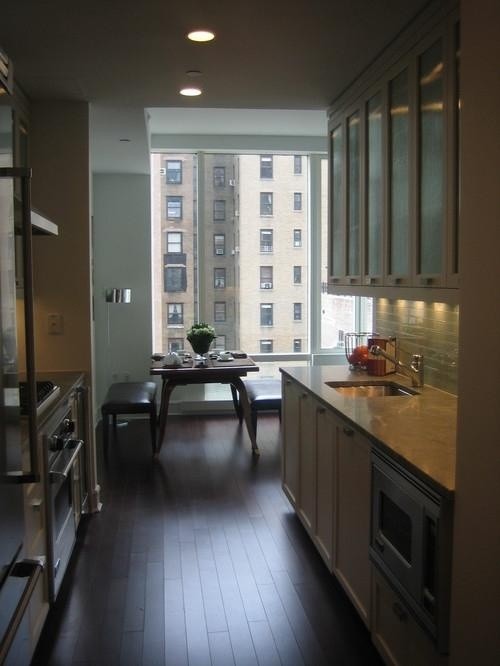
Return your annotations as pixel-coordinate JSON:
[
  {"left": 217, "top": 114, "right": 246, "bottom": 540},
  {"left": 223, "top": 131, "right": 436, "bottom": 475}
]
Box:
[{"left": 187, "top": 321, "right": 216, "bottom": 338}]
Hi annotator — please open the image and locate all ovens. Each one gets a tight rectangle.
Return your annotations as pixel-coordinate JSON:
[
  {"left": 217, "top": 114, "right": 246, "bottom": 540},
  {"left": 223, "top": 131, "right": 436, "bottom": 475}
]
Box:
[{"left": 30, "top": 401, "right": 85, "bottom": 607}]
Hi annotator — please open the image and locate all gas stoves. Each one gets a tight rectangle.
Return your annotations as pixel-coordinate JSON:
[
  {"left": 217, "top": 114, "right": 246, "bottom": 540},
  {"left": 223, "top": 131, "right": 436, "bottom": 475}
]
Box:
[{"left": 19, "top": 380, "right": 61, "bottom": 418}]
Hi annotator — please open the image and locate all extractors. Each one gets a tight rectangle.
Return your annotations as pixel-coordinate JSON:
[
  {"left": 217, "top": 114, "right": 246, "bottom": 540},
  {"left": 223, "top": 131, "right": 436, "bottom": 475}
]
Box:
[{"left": 15, "top": 194, "right": 59, "bottom": 237}]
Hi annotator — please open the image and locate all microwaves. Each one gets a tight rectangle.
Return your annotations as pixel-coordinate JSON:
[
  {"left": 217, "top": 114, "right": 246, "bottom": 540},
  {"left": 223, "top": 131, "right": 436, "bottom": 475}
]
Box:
[{"left": 364, "top": 446, "right": 442, "bottom": 642}]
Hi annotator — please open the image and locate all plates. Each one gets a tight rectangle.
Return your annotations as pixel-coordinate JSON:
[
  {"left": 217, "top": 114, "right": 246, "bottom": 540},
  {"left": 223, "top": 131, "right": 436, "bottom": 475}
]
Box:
[
  {"left": 151, "top": 354, "right": 166, "bottom": 361},
  {"left": 217, "top": 355, "right": 234, "bottom": 361}
]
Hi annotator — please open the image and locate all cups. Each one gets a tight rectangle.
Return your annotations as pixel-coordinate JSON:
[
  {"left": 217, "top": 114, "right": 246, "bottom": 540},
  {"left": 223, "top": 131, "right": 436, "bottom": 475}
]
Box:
[{"left": 219, "top": 352, "right": 231, "bottom": 360}]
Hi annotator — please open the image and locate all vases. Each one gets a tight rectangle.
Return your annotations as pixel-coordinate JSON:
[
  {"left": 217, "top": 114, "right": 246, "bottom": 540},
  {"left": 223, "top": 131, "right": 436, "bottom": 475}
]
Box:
[{"left": 187, "top": 338, "right": 217, "bottom": 356}]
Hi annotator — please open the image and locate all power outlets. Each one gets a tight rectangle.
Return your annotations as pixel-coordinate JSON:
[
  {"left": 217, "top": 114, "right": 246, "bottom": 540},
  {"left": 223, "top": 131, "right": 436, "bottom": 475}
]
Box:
[
  {"left": 49, "top": 314, "right": 63, "bottom": 334},
  {"left": 104, "top": 287, "right": 131, "bottom": 342}
]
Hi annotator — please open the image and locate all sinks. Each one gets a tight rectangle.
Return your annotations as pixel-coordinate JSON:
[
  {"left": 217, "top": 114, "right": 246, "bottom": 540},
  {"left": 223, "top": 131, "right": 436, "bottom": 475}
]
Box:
[{"left": 323, "top": 377, "right": 421, "bottom": 399}]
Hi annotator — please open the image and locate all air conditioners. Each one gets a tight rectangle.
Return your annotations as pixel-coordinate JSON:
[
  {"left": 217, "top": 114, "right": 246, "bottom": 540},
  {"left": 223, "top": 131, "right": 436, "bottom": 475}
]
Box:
[
  {"left": 229, "top": 180, "right": 236, "bottom": 186},
  {"left": 216, "top": 249, "right": 223, "bottom": 255}
]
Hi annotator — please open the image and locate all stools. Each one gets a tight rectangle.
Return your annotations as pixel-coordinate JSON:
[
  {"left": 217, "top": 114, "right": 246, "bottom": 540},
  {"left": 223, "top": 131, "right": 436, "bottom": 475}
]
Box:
[
  {"left": 237, "top": 379, "right": 282, "bottom": 446},
  {"left": 100, "top": 381, "right": 157, "bottom": 464}
]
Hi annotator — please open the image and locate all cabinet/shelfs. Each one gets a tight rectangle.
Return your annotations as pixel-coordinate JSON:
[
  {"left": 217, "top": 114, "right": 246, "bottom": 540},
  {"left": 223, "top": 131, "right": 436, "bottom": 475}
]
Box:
[
  {"left": 280, "top": 373, "right": 500, "bottom": 666},
  {"left": 328, "top": 0, "right": 500, "bottom": 295}
]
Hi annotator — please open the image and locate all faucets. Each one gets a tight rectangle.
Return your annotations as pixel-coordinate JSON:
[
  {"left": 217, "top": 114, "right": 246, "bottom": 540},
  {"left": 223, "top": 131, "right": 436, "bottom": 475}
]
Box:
[{"left": 368, "top": 343, "right": 425, "bottom": 386}]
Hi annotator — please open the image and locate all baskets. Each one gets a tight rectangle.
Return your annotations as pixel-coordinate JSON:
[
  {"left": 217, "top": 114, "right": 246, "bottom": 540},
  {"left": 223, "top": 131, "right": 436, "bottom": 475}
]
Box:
[{"left": 345, "top": 332, "right": 379, "bottom": 370}]
